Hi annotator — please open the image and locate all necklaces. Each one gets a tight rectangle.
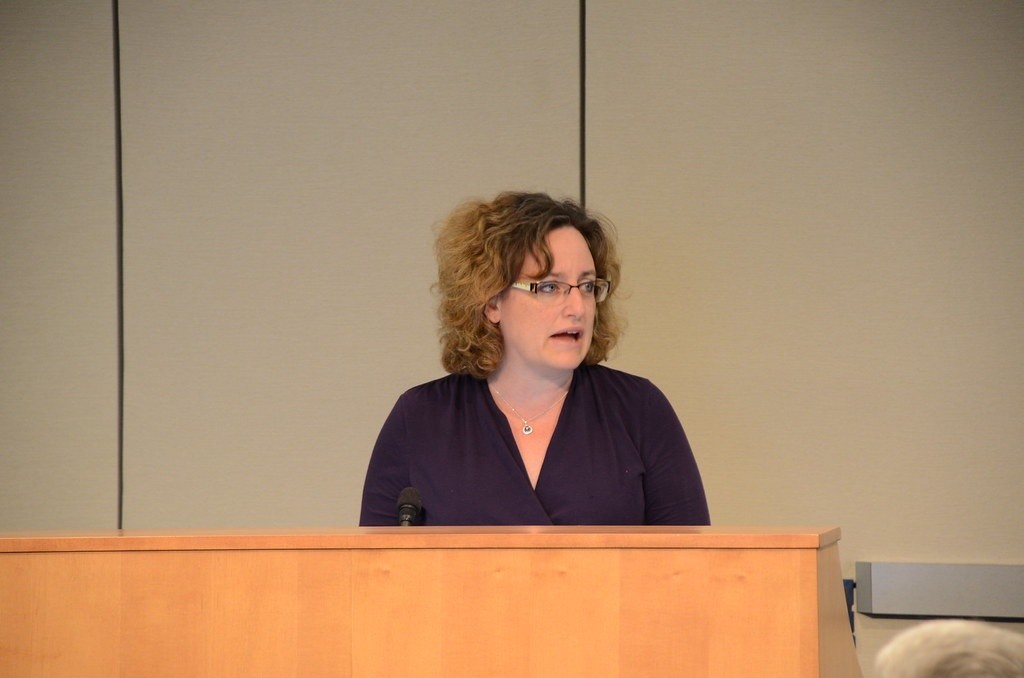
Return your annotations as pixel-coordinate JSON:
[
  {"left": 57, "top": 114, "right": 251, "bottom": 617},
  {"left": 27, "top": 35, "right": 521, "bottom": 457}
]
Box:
[{"left": 493, "top": 388, "right": 568, "bottom": 435}]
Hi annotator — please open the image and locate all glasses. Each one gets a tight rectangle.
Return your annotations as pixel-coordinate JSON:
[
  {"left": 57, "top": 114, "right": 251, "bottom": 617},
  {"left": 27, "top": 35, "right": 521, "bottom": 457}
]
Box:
[{"left": 511, "top": 278, "right": 611, "bottom": 307}]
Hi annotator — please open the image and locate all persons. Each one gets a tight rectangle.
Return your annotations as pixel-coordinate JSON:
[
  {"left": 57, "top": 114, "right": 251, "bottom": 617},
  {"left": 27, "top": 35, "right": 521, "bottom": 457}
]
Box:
[{"left": 358, "top": 190, "right": 711, "bottom": 526}]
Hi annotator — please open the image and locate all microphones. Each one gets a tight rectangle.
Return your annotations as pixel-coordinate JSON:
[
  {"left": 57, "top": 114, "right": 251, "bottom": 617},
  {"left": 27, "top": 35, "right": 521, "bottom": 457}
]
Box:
[{"left": 397, "top": 487, "right": 422, "bottom": 525}]
